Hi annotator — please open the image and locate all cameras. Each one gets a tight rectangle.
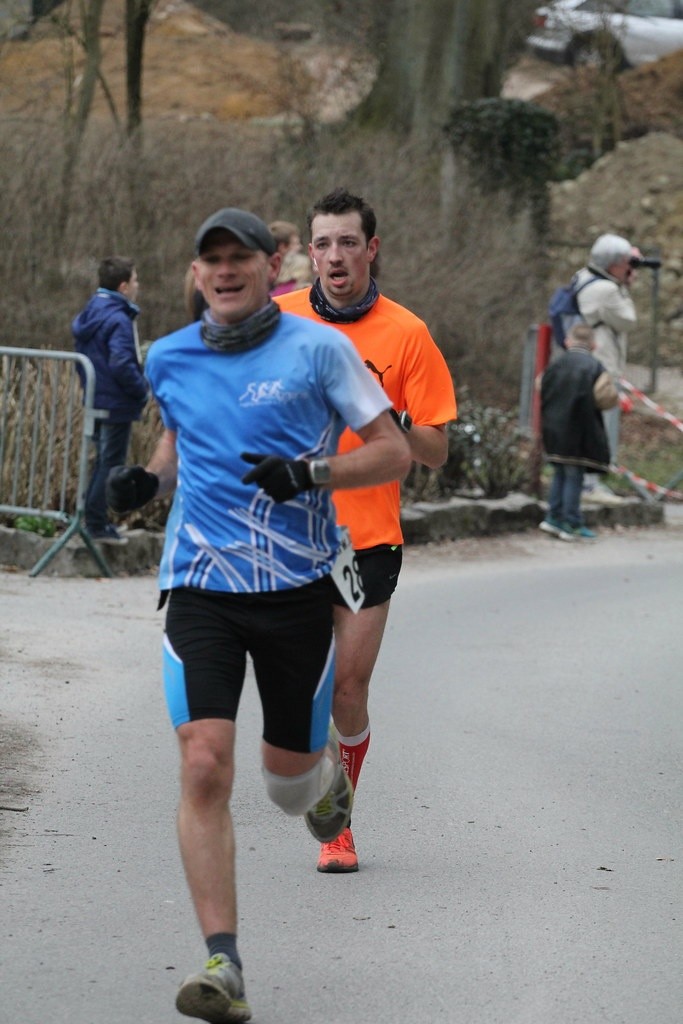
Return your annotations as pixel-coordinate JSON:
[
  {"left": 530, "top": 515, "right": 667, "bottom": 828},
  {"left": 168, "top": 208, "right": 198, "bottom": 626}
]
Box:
[{"left": 629, "top": 257, "right": 662, "bottom": 270}]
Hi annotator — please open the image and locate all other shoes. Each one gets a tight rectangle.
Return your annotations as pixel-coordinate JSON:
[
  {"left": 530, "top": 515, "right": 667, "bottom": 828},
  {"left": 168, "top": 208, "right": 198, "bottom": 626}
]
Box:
[{"left": 583, "top": 479, "right": 617, "bottom": 499}]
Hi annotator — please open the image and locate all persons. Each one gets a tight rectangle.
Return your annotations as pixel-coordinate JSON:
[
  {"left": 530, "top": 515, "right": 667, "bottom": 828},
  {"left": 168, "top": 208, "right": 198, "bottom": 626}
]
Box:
[
  {"left": 548, "top": 234, "right": 644, "bottom": 507},
  {"left": 72, "top": 257, "right": 150, "bottom": 546},
  {"left": 105, "top": 208, "right": 411, "bottom": 1017},
  {"left": 272, "top": 188, "right": 458, "bottom": 1023},
  {"left": 534, "top": 323, "right": 618, "bottom": 543},
  {"left": 183, "top": 221, "right": 309, "bottom": 324}
]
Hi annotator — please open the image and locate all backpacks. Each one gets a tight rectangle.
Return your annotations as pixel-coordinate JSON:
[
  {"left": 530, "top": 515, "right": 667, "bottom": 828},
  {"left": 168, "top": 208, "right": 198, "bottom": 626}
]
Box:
[{"left": 548, "top": 268, "right": 603, "bottom": 350}]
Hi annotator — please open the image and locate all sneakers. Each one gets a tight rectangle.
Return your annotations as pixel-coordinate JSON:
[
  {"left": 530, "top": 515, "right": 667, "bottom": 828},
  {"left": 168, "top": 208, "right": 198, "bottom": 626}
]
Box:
[
  {"left": 176, "top": 952, "right": 251, "bottom": 1024},
  {"left": 539, "top": 514, "right": 562, "bottom": 537},
  {"left": 303, "top": 719, "right": 354, "bottom": 843},
  {"left": 84, "top": 520, "right": 129, "bottom": 547},
  {"left": 316, "top": 828, "right": 358, "bottom": 872},
  {"left": 559, "top": 522, "right": 600, "bottom": 544}
]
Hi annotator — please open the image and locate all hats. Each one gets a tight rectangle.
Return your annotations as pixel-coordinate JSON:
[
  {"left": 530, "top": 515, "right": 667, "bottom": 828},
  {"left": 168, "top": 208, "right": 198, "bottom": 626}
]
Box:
[
  {"left": 590, "top": 234, "right": 632, "bottom": 270},
  {"left": 195, "top": 207, "right": 276, "bottom": 255}
]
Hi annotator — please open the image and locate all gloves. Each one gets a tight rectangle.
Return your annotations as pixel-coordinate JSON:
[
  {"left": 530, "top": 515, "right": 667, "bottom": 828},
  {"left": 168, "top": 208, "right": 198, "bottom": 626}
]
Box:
[
  {"left": 240, "top": 452, "right": 315, "bottom": 504},
  {"left": 105, "top": 465, "right": 159, "bottom": 513}
]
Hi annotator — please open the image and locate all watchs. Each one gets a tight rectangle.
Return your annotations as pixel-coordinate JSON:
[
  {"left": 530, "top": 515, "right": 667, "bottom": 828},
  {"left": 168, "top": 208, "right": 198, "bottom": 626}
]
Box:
[
  {"left": 302, "top": 459, "right": 332, "bottom": 489},
  {"left": 399, "top": 412, "right": 412, "bottom": 434}
]
